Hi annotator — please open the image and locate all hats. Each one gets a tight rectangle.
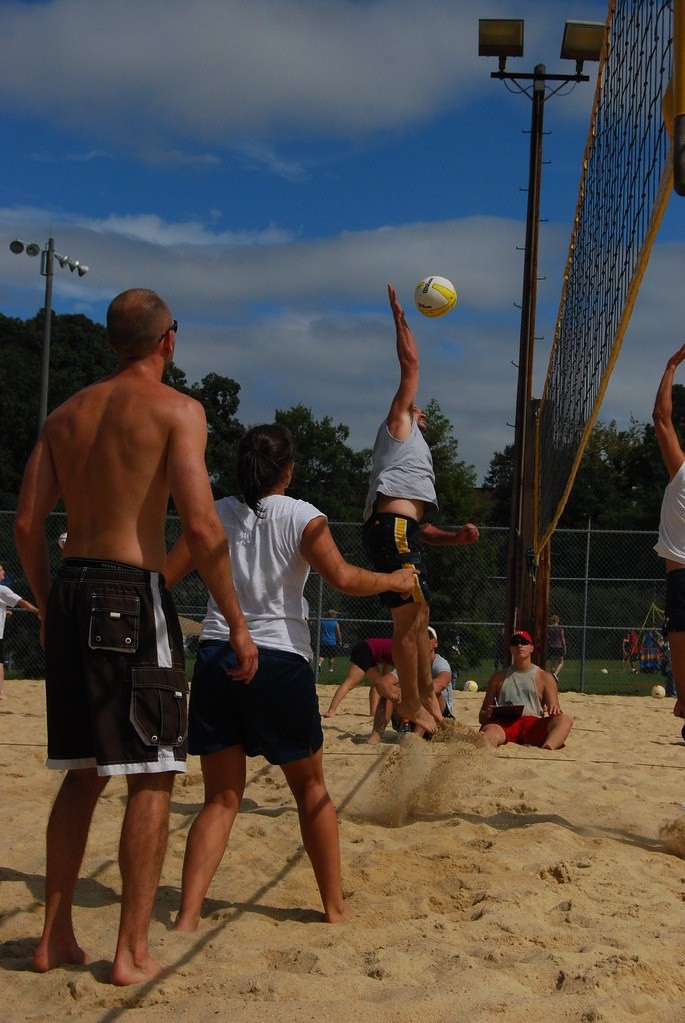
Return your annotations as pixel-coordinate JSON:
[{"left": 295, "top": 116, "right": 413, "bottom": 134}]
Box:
[
  {"left": 428, "top": 626, "right": 437, "bottom": 641},
  {"left": 328, "top": 610, "right": 338, "bottom": 615},
  {"left": 510, "top": 631, "right": 532, "bottom": 644}
]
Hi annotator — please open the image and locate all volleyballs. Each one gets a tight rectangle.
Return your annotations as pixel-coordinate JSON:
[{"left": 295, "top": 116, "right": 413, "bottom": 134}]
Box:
[
  {"left": 651, "top": 684, "right": 665, "bottom": 700},
  {"left": 464, "top": 679, "right": 478, "bottom": 694},
  {"left": 414, "top": 274, "right": 458, "bottom": 319}
]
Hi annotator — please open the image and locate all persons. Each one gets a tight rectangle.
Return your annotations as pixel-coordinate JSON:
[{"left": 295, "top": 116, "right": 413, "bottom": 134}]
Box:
[
  {"left": 362, "top": 284, "right": 478, "bottom": 735},
  {"left": 324, "top": 626, "right": 455, "bottom": 745},
  {"left": 546, "top": 614, "right": 566, "bottom": 683},
  {"left": 319, "top": 610, "right": 342, "bottom": 673},
  {"left": 623, "top": 633, "right": 635, "bottom": 672},
  {"left": 479, "top": 630, "right": 573, "bottom": 751},
  {"left": 652, "top": 343, "right": 685, "bottom": 743},
  {"left": 0, "top": 288, "right": 421, "bottom": 986}
]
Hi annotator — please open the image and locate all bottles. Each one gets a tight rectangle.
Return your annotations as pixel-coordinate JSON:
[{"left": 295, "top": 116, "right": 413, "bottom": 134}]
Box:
[{"left": 398, "top": 719, "right": 410, "bottom": 743}]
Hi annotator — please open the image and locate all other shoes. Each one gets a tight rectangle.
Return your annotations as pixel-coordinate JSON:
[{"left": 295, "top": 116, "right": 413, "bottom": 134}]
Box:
[{"left": 552, "top": 672, "right": 559, "bottom": 682}]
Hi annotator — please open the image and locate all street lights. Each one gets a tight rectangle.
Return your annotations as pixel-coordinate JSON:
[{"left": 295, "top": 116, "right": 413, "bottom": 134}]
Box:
[
  {"left": 477, "top": 15, "right": 609, "bottom": 634},
  {"left": 8, "top": 238, "right": 89, "bottom": 442}
]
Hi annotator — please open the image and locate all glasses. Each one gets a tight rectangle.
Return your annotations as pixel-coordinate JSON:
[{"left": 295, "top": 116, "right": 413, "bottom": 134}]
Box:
[
  {"left": 512, "top": 640, "right": 530, "bottom": 645},
  {"left": 159, "top": 320, "right": 177, "bottom": 342}
]
